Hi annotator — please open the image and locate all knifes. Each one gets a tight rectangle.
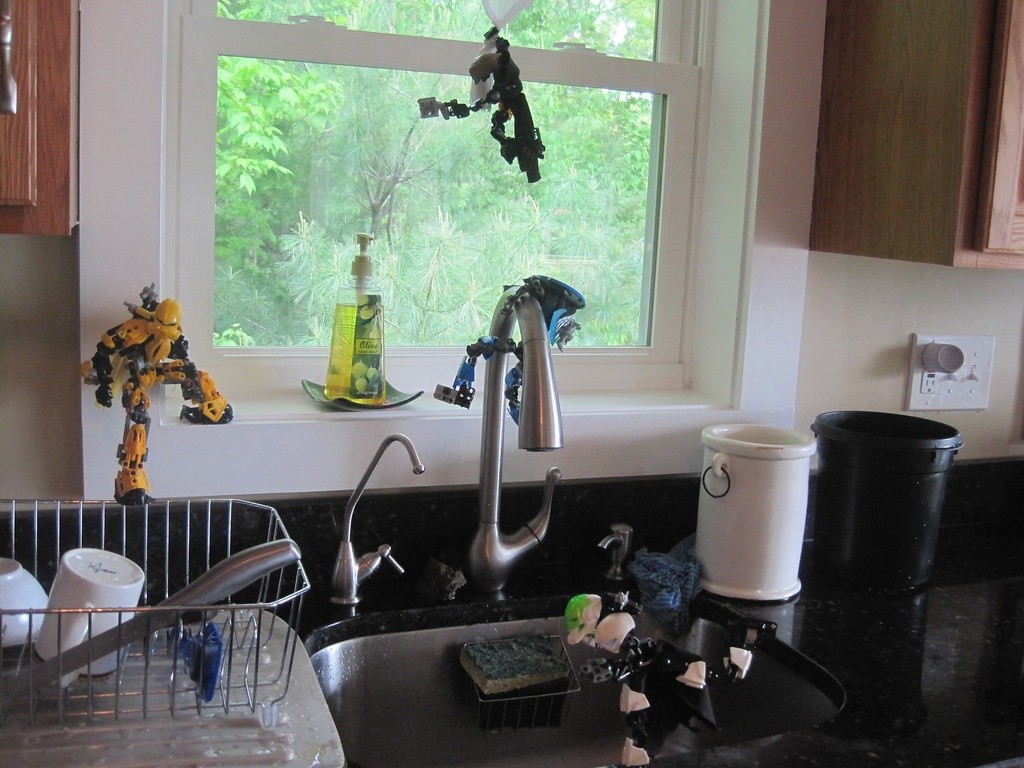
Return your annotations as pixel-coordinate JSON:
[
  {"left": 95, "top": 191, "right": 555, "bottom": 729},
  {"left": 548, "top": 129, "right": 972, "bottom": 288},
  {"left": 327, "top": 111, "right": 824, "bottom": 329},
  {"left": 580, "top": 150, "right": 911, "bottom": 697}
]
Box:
[{"left": 0, "top": 538, "right": 302, "bottom": 703}]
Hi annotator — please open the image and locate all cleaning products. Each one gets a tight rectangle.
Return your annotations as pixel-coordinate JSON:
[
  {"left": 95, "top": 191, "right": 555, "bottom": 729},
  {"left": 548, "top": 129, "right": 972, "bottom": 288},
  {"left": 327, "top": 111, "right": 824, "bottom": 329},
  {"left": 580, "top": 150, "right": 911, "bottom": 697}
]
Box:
[{"left": 322, "top": 235, "right": 387, "bottom": 408}]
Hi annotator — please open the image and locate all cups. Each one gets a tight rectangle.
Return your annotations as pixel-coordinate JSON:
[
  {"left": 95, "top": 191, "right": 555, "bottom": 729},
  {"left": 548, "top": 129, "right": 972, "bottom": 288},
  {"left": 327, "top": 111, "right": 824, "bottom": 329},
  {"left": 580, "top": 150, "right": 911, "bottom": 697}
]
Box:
[{"left": 36, "top": 548, "right": 145, "bottom": 675}]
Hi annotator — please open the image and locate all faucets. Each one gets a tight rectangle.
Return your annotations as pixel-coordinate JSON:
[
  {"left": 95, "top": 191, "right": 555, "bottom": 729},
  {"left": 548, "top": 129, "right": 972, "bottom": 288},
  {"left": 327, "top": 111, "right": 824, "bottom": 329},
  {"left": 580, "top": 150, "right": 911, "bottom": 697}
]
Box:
[
  {"left": 467, "top": 281, "right": 567, "bottom": 605},
  {"left": 328, "top": 434, "right": 425, "bottom": 605}
]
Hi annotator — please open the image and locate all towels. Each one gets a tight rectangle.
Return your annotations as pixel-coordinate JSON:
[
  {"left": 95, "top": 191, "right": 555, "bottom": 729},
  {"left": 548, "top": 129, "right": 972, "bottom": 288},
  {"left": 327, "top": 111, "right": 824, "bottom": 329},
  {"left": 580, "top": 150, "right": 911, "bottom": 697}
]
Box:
[{"left": 621, "top": 543, "right": 705, "bottom": 641}]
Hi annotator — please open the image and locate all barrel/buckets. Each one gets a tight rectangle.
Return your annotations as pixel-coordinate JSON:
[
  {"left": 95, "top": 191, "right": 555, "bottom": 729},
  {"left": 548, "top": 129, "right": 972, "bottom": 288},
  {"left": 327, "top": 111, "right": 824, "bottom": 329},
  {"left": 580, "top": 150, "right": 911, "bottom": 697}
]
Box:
[
  {"left": 695, "top": 423, "right": 817, "bottom": 600},
  {"left": 809, "top": 410, "right": 965, "bottom": 587}
]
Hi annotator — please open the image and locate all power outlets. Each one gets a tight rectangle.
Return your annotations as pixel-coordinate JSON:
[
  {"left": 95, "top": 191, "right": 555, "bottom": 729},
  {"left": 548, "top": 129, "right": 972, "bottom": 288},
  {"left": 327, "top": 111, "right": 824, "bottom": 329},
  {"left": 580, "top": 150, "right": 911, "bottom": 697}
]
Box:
[{"left": 905, "top": 331, "right": 996, "bottom": 412}]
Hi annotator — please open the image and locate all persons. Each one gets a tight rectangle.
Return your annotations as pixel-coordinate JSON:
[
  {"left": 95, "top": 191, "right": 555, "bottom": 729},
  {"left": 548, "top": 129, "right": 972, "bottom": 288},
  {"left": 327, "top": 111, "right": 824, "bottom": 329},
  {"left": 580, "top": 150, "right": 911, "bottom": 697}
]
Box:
[
  {"left": 80, "top": 283, "right": 233, "bottom": 504},
  {"left": 434, "top": 275, "right": 585, "bottom": 426},
  {"left": 565, "top": 593, "right": 777, "bottom": 768}
]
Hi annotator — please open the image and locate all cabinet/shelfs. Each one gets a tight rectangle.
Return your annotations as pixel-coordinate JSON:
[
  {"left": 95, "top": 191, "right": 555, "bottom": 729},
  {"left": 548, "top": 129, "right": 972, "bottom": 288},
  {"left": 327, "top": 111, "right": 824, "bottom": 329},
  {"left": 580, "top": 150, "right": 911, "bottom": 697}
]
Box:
[
  {"left": 0, "top": 0, "right": 80, "bottom": 239},
  {"left": 810, "top": 0, "right": 1024, "bottom": 270}
]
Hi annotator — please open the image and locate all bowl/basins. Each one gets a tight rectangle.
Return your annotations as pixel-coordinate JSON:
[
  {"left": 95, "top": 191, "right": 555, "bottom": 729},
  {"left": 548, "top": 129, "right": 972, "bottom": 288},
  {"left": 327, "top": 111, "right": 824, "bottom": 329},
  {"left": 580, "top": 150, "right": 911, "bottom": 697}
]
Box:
[{"left": 0, "top": 558, "right": 48, "bottom": 647}]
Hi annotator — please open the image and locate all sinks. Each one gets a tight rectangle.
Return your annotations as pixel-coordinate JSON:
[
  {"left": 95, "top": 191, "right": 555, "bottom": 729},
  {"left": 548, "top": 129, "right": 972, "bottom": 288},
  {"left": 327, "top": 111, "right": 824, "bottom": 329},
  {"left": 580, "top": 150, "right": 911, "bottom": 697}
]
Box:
[{"left": 298, "top": 597, "right": 850, "bottom": 768}]
